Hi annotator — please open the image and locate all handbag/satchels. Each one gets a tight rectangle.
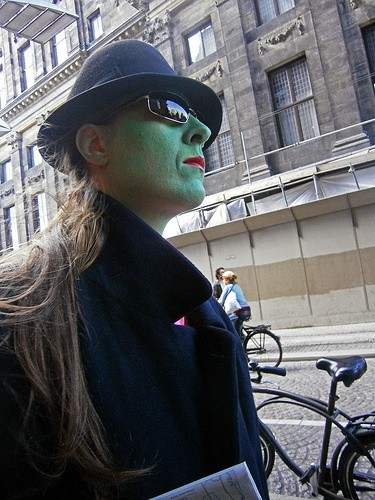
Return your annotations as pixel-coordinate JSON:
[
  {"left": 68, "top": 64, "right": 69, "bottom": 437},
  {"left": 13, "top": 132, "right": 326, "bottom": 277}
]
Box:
[{"left": 224, "top": 290, "right": 242, "bottom": 315}]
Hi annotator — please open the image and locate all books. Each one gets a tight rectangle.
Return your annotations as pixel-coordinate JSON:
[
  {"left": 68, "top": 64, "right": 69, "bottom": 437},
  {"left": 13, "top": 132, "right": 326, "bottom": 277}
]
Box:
[{"left": 148, "top": 461, "right": 262, "bottom": 500}]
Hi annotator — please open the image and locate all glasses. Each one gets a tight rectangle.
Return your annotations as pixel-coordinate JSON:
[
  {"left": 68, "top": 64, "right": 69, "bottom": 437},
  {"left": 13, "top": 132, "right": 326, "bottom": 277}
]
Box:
[{"left": 94, "top": 89, "right": 212, "bottom": 152}]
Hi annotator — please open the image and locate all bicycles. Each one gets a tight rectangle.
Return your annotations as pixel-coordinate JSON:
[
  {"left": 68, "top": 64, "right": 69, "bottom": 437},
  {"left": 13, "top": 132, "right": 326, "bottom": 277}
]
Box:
[
  {"left": 227, "top": 314, "right": 283, "bottom": 368},
  {"left": 247, "top": 355, "right": 375, "bottom": 500}
]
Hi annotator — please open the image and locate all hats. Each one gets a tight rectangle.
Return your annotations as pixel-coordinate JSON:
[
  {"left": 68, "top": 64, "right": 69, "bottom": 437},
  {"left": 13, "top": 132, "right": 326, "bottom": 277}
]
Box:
[{"left": 37, "top": 39, "right": 223, "bottom": 177}]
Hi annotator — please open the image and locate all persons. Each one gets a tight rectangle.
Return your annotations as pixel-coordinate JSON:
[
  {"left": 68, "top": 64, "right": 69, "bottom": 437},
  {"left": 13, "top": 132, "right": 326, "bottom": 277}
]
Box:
[
  {"left": 212, "top": 267, "right": 225, "bottom": 300},
  {"left": 219, "top": 270, "right": 248, "bottom": 343},
  {"left": 0, "top": 41, "right": 268, "bottom": 500}
]
